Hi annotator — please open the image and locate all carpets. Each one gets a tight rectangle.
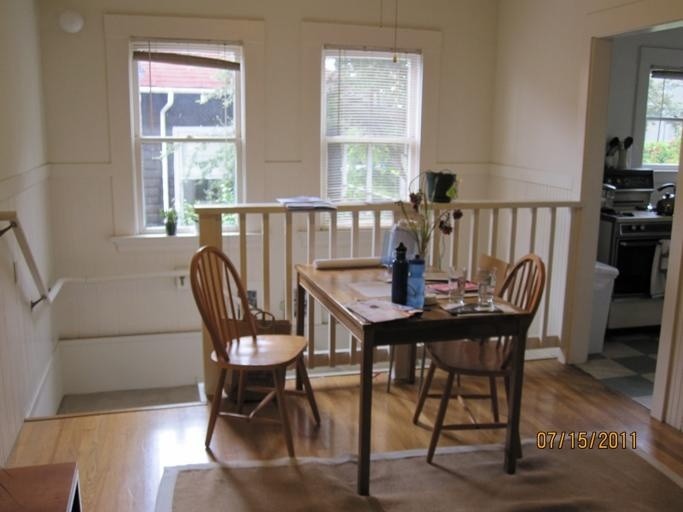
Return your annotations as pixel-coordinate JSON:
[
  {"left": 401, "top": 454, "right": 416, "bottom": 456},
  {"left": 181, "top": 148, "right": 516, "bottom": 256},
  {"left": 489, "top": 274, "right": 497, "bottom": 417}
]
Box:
[{"left": 154, "top": 429, "right": 683, "bottom": 511}]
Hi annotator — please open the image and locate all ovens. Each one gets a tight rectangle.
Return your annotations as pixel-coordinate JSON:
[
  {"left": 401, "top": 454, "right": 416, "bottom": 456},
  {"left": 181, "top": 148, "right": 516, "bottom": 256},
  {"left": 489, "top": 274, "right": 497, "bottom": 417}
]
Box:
[{"left": 610, "top": 221, "right": 676, "bottom": 302}]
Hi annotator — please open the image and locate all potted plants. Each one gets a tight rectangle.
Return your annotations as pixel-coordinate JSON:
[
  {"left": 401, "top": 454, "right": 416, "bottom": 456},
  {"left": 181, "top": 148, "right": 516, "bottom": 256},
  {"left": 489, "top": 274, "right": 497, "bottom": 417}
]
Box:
[
  {"left": 159, "top": 209, "right": 178, "bottom": 235},
  {"left": 409, "top": 169, "right": 461, "bottom": 203}
]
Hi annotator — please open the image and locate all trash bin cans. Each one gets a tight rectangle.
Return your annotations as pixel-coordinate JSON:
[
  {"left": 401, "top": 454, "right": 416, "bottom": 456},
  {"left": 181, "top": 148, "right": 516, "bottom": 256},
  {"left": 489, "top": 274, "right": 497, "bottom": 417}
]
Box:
[{"left": 588, "top": 262, "right": 619, "bottom": 354}]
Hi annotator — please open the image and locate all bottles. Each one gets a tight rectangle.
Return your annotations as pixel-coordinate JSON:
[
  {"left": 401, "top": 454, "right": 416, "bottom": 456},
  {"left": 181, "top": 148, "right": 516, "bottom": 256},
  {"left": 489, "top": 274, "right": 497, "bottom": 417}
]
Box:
[{"left": 390, "top": 241, "right": 426, "bottom": 310}]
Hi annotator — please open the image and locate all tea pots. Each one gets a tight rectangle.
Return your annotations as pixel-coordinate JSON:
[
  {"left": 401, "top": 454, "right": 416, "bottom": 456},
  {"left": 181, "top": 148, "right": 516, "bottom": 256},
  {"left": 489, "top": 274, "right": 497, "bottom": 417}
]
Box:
[{"left": 656, "top": 182, "right": 675, "bottom": 216}]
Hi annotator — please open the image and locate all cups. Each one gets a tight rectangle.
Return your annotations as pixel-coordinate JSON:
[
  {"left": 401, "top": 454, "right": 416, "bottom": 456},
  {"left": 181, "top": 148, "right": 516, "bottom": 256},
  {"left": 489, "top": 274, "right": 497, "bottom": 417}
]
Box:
[
  {"left": 446, "top": 264, "right": 468, "bottom": 305},
  {"left": 475, "top": 263, "right": 498, "bottom": 309},
  {"left": 617, "top": 149, "right": 631, "bottom": 169}
]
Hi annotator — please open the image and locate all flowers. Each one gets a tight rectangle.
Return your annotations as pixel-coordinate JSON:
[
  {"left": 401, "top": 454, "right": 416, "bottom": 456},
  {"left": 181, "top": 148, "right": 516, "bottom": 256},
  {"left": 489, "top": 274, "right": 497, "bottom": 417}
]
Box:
[{"left": 394, "top": 191, "right": 463, "bottom": 258}]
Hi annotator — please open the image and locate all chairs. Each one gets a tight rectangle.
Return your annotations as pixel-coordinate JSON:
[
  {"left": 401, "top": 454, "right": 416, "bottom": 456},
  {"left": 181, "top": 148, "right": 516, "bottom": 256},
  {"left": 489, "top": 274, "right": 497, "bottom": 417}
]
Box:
[
  {"left": 190, "top": 244, "right": 320, "bottom": 459},
  {"left": 414, "top": 254, "right": 545, "bottom": 462}
]
